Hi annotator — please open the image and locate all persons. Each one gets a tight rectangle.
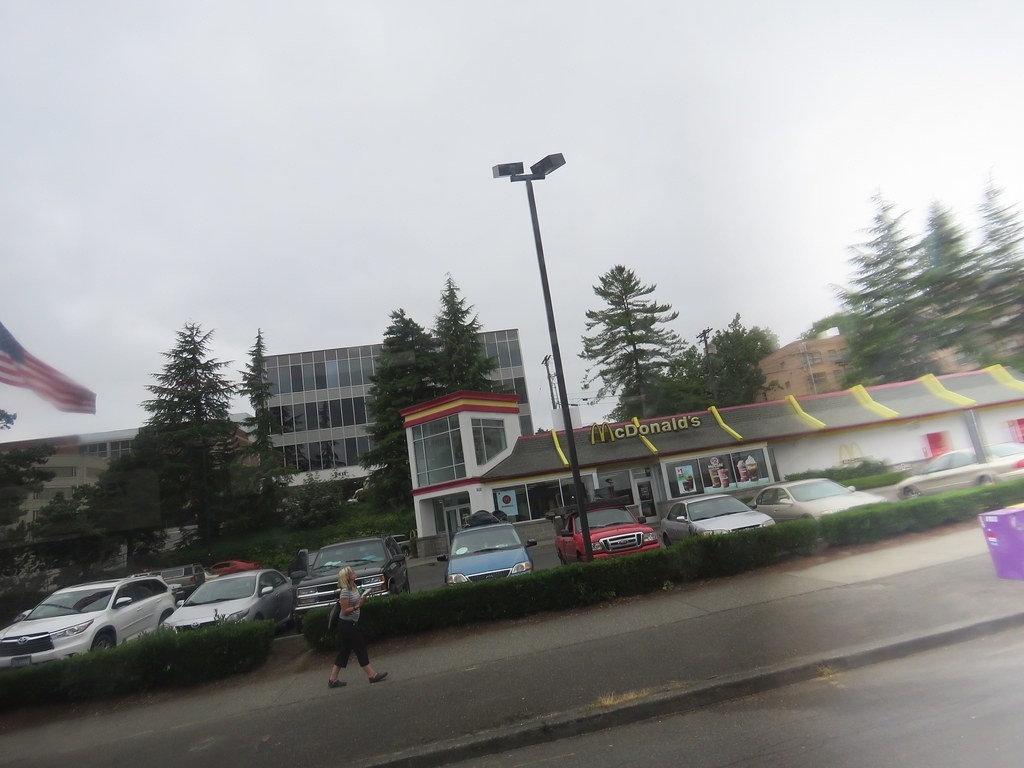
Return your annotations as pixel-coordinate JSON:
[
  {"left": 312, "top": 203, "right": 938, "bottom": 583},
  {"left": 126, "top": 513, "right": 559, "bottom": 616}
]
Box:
[{"left": 326, "top": 565, "right": 389, "bottom": 687}]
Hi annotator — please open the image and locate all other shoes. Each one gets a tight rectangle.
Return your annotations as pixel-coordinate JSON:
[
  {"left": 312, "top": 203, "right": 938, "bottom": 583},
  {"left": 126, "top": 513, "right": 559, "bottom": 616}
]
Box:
[
  {"left": 328, "top": 678, "right": 347, "bottom": 688},
  {"left": 368, "top": 670, "right": 389, "bottom": 684}
]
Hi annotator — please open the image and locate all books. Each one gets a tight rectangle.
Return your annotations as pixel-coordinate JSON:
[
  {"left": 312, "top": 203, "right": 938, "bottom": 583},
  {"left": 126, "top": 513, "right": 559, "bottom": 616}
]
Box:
[{"left": 359, "top": 588, "right": 373, "bottom": 600}]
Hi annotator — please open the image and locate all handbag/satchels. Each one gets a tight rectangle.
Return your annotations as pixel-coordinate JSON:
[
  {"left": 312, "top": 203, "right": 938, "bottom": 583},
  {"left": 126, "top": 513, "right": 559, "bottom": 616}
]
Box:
[{"left": 326, "top": 602, "right": 343, "bottom": 630}]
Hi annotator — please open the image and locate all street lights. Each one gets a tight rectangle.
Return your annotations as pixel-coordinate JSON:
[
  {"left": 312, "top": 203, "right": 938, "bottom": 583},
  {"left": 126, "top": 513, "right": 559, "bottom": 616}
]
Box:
[{"left": 491, "top": 150, "right": 599, "bottom": 567}]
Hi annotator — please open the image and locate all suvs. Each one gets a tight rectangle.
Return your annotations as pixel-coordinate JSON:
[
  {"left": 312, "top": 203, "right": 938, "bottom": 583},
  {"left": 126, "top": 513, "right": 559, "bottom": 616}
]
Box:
[
  {"left": 544, "top": 496, "right": 660, "bottom": 566},
  {"left": 388, "top": 534, "right": 412, "bottom": 558}
]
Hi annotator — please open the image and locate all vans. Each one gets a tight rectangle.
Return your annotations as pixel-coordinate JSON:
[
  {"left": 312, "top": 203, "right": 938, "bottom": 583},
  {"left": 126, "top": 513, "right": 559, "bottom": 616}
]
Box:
[{"left": 120, "top": 561, "right": 206, "bottom": 601}]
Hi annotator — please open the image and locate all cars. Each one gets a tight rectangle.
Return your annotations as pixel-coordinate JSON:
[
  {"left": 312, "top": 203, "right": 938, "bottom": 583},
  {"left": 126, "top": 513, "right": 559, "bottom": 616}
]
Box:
[
  {"left": 435, "top": 521, "right": 538, "bottom": 587},
  {"left": 658, "top": 492, "right": 776, "bottom": 549},
  {"left": 156, "top": 568, "right": 293, "bottom": 638},
  {"left": 0, "top": 576, "right": 179, "bottom": 670},
  {"left": 746, "top": 477, "right": 886, "bottom": 520},
  {"left": 210, "top": 559, "right": 259, "bottom": 575},
  {"left": 895, "top": 442, "right": 1023, "bottom": 501},
  {"left": 290, "top": 533, "right": 411, "bottom": 632}
]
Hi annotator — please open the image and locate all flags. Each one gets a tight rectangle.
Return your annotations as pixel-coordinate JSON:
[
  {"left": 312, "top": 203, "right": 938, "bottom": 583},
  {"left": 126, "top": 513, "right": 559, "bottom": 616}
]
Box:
[{"left": 0, "top": 321, "right": 97, "bottom": 417}]
